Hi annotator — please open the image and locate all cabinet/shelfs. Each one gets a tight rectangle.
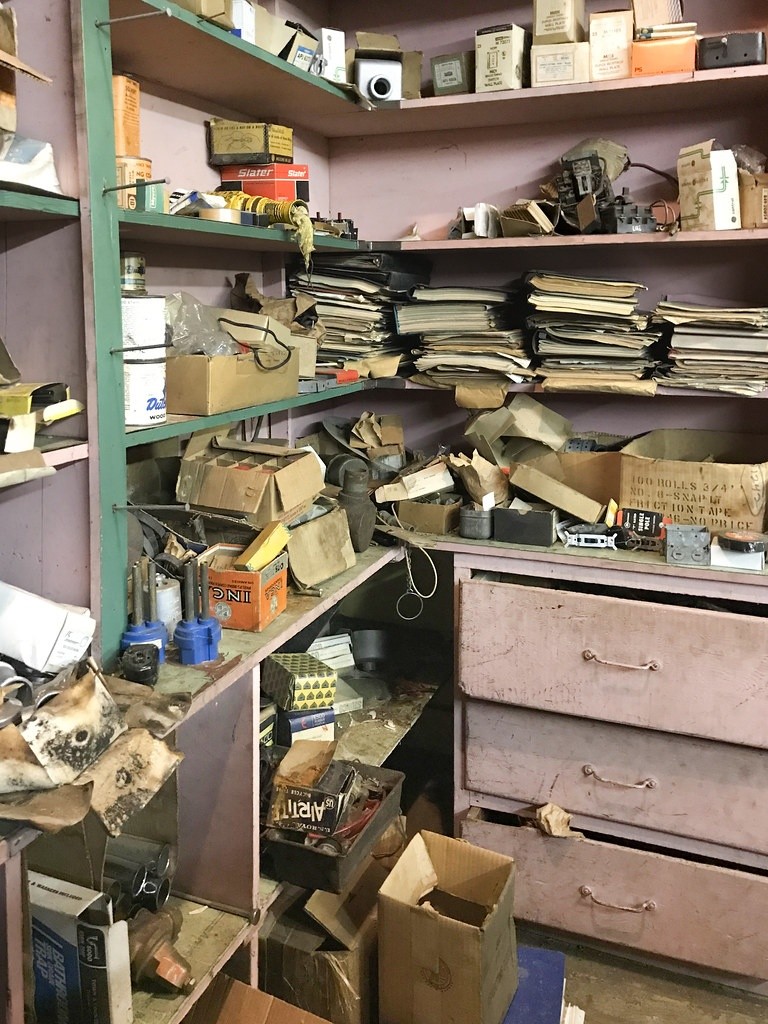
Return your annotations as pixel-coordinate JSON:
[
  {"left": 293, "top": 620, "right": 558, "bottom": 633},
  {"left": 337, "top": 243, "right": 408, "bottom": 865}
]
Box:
[
  {"left": 2, "top": 1, "right": 433, "bottom": 1024},
  {"left": 357, "top": 1, "right": 767, "bottom": 1010}
]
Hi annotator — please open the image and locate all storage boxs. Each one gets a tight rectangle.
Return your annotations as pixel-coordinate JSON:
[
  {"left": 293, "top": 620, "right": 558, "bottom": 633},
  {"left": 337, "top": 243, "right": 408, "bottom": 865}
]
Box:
[
  {"left": 175, "top": 425, "right": 326, "bottom": 530},
  {"left": 269, "top": 810, "right": 407, "bottom": 1024},
  {"left": 166, "top": 347, "right": 301, "bottom": 415},
  {"left": 377, "top": 829, "right": 519, "bottom": 1024},
  {"left": 286, "top": 508, "right": 357, "bottom": 594},
  {"left": 620, "top": 427, "right": 768, "bottom": 534},
  {"left": 192, "top": 541, "right": 288, "bottom": 632},
  {"left": 520, "top": 431, "right": 626, "bottom": 508}
]
